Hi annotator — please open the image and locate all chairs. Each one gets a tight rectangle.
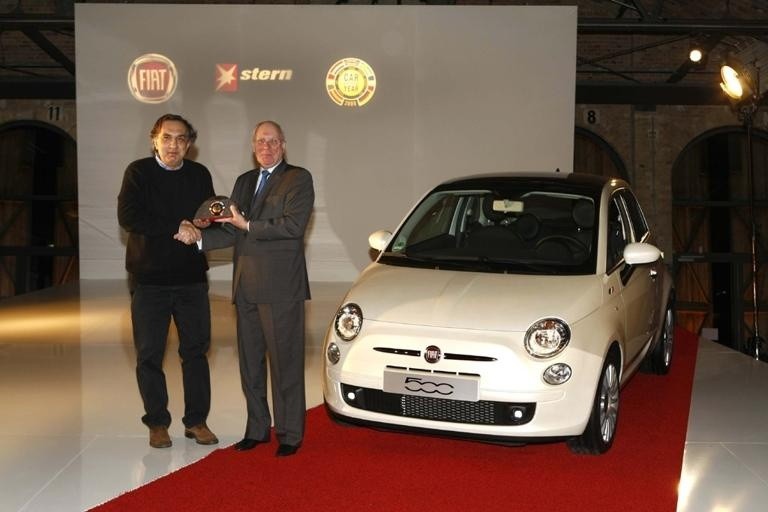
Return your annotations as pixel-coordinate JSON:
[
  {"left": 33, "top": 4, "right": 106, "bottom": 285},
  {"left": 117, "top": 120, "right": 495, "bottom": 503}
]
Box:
[{"left": 463, "top": 198, "right": 617, "bottom": 266}]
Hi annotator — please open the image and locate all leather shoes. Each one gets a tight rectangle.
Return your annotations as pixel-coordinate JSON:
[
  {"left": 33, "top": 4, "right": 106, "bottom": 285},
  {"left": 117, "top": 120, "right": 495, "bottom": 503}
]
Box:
[
  {"left": 149, "top": 426, "right": 172, "bottom": 448},
  {"left": 185, "top": 423, "right": 218, "bottom": 444},
  {"left": 235, "top": 439, "right": 258, "bottom": 449},
  {"left": 278, "top": 445, "right": 297, "bottom": 456}
]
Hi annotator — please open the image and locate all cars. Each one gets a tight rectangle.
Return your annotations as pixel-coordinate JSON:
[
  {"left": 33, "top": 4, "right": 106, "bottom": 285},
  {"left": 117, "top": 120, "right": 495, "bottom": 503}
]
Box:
[{"left": 318, "top": 166, "right": 681, "bottom": 458}]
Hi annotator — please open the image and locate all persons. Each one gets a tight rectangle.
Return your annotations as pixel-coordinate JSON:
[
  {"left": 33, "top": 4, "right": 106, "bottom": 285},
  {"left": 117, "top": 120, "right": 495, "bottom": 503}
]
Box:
[
  {"left": 118, "top": 114, "right": 218, "bottom": 448},
  {"left": 174, "top": 121, "right": 314, "bottom": 457}
]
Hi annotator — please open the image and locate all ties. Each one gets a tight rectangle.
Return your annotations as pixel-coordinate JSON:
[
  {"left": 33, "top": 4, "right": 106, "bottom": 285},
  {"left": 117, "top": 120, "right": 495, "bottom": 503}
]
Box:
[{"left": 252, "top": 170, "right": 270, "bottom": 207}]
